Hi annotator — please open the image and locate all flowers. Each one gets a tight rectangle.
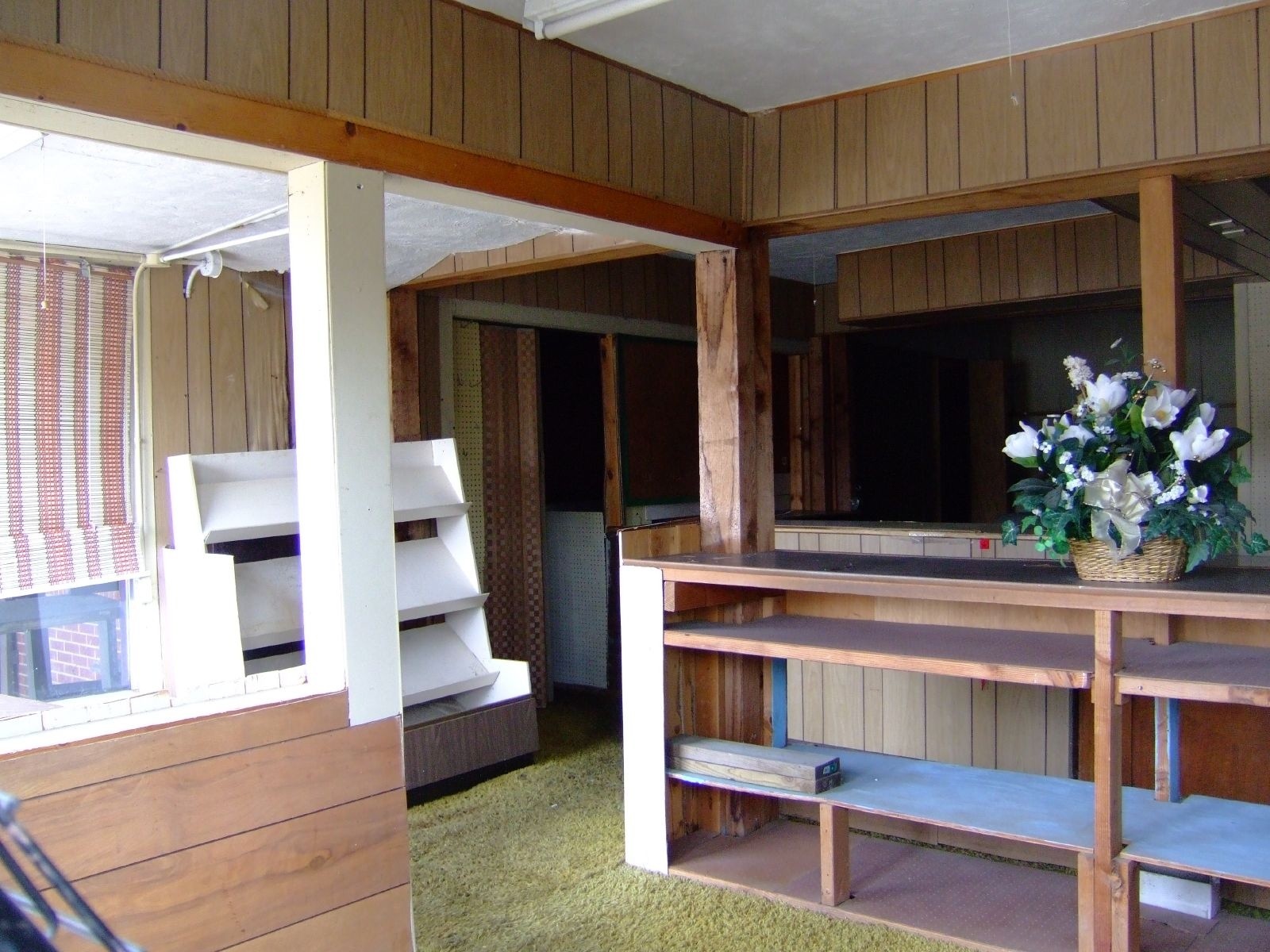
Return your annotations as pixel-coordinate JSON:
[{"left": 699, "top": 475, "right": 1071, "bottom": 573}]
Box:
[{"left": 1001, "top": 353, "right": 1270, "bottom": 573}]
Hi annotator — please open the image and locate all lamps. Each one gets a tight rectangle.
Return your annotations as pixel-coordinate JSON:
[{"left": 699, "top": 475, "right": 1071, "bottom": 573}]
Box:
[{"left": 523, "top": 0, "right": 672, "bottom": 41}]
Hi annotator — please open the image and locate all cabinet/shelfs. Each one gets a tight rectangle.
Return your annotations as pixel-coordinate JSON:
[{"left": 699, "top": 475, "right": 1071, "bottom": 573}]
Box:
[
  {"left": 616, "top": 517, "right": 1270, "bottom": 952},
  {"left": 166, "top": 437, "right": 538, "bottom": 789}
]
